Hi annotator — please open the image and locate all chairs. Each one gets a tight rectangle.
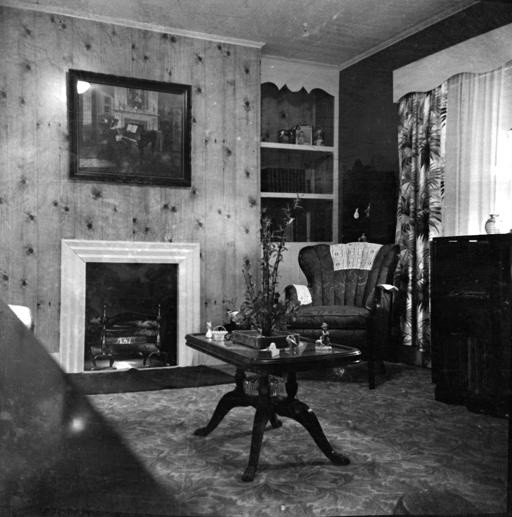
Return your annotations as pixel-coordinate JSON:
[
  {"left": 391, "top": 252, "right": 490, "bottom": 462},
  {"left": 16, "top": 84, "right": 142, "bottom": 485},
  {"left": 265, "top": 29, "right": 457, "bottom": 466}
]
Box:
[{"left": 283, "top": 240, "right": 401, "bottom": 388}]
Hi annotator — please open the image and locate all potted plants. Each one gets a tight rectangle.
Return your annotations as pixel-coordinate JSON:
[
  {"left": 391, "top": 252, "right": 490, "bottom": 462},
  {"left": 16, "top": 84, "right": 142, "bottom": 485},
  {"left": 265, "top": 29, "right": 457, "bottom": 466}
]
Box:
[{"left": 232, "top": 191, "right": 304, "bottom": 349}]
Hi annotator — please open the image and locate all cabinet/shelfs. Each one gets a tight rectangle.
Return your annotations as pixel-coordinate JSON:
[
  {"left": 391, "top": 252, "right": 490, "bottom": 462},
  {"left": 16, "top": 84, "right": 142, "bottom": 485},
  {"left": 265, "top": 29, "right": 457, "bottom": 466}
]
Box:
[
  {"left": 429, "top": 231, "right": 512, "bottom": 418},
  {"left": 260, "top": 55, "right": 340, "bottom": 292}
]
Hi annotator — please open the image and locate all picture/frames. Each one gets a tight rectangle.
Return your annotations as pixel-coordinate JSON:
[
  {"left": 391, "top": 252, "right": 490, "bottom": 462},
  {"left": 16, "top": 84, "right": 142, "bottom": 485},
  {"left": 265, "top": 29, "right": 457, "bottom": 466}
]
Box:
[{"left": 68, "top": 67, "right": 192, "bottom": 189}]
